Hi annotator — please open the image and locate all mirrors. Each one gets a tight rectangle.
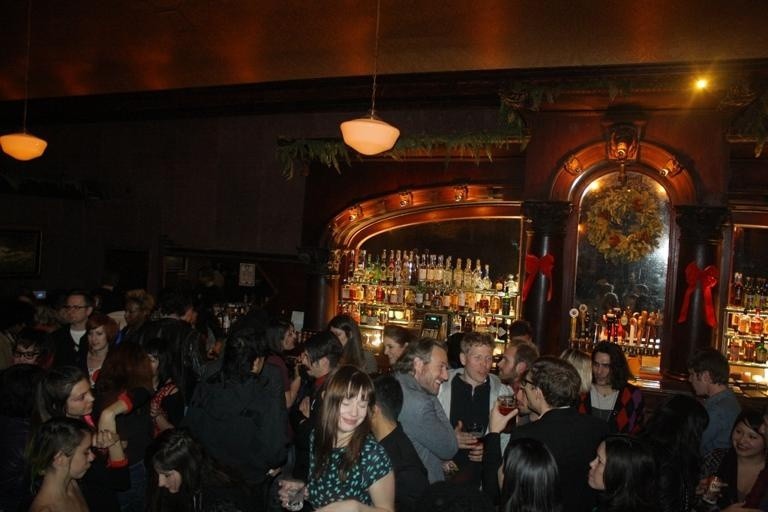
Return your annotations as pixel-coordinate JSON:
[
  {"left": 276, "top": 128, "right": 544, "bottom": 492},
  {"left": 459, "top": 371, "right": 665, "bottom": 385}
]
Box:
[{"left": 575, "top": 175, "right": 669, "bottom": 351}]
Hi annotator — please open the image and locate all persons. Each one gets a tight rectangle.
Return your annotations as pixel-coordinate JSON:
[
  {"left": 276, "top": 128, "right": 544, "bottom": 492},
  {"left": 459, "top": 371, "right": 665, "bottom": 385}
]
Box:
[
  {"left": 695, "top": 409, "right": 768, "bottom": 512},
  {"left": 292, "top": 330, "right": 348, "bottom": 431},
  {"left": 29, "top": 416, "right": 95, "bottom": 512},
  {"left": 498, "top": 341, "right": 538, "bottom": 390},
  {"left": 328, "top": 315, "right": 378, "bottom": 373},
  {"left": 186, "top": 303, "right": 227, "bottom": 404},
  {"left": 14, "top": 328, "right": 57, "bottom": 369},
  {"left": 437, "top": 332, "right": 518, "bottom": 489},
  {"left": 634, "top": 394, "right": 762, "bottom": 512},
  {"left": 500, "top": 438, "right": 562, "bottom": 512},
  {"left": 384, "top": 325, "right": 413, "bottom": 366},
  {"left": 577, "top": 342, "right": 649, "bottom": 439},
  {"left": 0, "top": 363, "right": 50, "bottom": 512},
  {"left": 688, "top": 348, "right": 741, "bottom": 450},
  {"left": 138, "top": 285, "right": 193, "bottom": 375},
  {"left": 177, "top": 327, "right": 289, "bottom": 485},
  {"left": 142, "top": 331, "right": 184, "bottom": 437},
  {"left": 144, "top": 427, "right": 252, "bottom": 512},
  {"left": 368, "top": 373, "right": 430, "bottom": 512},
  {"left": 587, "top": 435, "right": 644, "bottom": 512},
  {"left": 591, "top": 269, "right": 650, "bottom": 309},
  {"left": 76, "top": 316, "right": 119, "bottom": 388},
  {"left": 638, "top": 393, "right": 727, "bottom": 512},
  {"left": 278, "top": 365, "right": 396, "bottom": 512},
  {"left": 113, "top": 290, "right": 154, "bottom": 345},
  {"left": 510, "top": 320, "right": 534, "bottom": 341},
  {"left": 23, "top": 365, "right": 150, "bottom": 512},
  {"left": 505, "top": 356, "right": 612, "bottom": 512},
  {"left": 395, "top": 338, "right": 458, "bottom": 484},
  {"left": 265, "top": 318, "right": 301, "bottom": 411},
  {"left": 52, "top": 290, "right": 97, "bottom": 370}
]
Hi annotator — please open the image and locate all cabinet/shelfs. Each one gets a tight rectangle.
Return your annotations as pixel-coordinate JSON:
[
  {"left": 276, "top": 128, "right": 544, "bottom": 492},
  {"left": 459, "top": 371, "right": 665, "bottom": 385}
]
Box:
[
  {"left": 713, "top": 137, "right": 768, "bottom": 408},
  {"left": 311, "top": 180, "right": 524, "bottom": 350}
]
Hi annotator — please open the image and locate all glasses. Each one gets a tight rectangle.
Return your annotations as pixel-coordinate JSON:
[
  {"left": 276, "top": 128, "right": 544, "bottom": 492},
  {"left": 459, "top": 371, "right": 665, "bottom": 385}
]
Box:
[
  {"left": 66, "top": 304, "right": 88, "bottom": 311},
  {"left": 13, "top": 350, "right": 39, "bottom": 360},
  {"left": 520, "top": 376, "right": 537, "bottom": 387}
]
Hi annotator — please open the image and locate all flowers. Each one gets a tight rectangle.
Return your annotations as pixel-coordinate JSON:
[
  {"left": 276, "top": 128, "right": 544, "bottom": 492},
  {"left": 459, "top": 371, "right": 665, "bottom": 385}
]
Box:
[{"left": 586, "top": 185, "right": 665, "bottom": 264}]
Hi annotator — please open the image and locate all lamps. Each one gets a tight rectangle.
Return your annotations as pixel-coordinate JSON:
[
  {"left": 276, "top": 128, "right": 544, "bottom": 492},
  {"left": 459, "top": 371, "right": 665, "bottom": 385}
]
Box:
[
  {"left": 0, "top": 1, "right": 49, "bottom": 163},
  {"left": 339, "top": 1, "right": 401, "bottom": 156}
]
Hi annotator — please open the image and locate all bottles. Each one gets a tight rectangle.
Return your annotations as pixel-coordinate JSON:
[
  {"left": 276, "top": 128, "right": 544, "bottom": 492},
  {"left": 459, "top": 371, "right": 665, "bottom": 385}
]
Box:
[
  {"left": 755, "top": 335, "right": 767, "bottom": 363},
  {"left": 490, "top": 289, "right": 500, "bottom": 314},
  {"left": 734, "top": 274, "right": 768, "bottom": 309},
  {"left": 341, "top": 279, "right": 476, "bottom": 314},
  {"left": 763, "top": 316, "right": 768, "bottom": 336},
  {"left": 342, "top": 303, "right": 378, "bottom": 326},
  {"left": 729, "top": 340, "right": 739, "bottom": 362},
  {"left": 502, "top": 286, "right": 510, "bottom": 315},
  {"left": 702, "top": 475, "right": 722, "bottom": 505},
  {"left": 744, "top": 339, "right": 754, "bottom": 362},
  {"left": 348, "top": 249, "right": 492, "bottom": 290},
  {"left": 750, "top": 308, "right": 762, "bottom": 336},
  {"left": 498, "top": 318, "right": 508, "bottom": 340},
  {"left": 738, "top": 309, "right": 750, "bottom": 335},
  {"left": 487, "top": 315, "right": 497, "bottom": 339},
  {"left": 479, "top": 289, "right": 489, "bottom": 314}
]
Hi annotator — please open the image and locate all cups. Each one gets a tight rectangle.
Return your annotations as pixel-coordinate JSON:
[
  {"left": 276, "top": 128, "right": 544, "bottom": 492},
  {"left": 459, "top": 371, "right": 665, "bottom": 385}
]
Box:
[
  {"left": 468, "top": 424, "right": 484, "bottom": 444},
  {"left": 288, "top": 480, "right": 304, "bottom": 511},
  {"left": 498, "top": 395, "right": 515, "bottom": 415}
]
